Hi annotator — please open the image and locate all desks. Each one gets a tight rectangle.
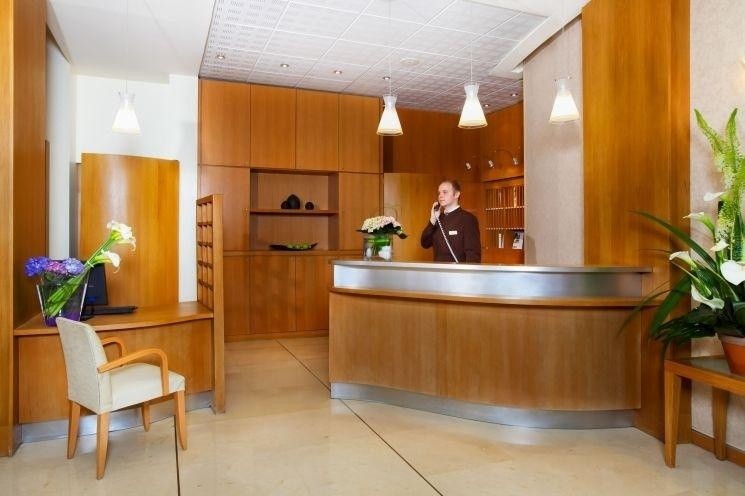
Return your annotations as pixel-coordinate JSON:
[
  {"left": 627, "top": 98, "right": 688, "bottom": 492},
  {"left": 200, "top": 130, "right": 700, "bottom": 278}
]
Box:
[
  {"left": 661, "top": 353, "right": 744, "bottom": 468},
  {"left": 13, "top": 299, "right": 214, "bottom": 443}
]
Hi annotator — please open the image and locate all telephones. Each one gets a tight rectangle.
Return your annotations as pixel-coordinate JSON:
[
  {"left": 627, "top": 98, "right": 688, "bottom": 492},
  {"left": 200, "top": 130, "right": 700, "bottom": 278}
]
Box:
[{"left": 434, "top": 202, "right": 440, "bottom": 211}]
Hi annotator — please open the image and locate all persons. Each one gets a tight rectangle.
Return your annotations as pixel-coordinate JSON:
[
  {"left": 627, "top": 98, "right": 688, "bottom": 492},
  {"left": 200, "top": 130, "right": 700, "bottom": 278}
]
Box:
[{"left": 420, "top": 180, "right": 481, "bottom": 263}]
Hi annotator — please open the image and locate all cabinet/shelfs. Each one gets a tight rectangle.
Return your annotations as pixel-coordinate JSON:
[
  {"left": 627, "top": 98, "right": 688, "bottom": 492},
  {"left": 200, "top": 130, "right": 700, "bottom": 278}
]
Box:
[
  {"left": 481, "top": 175, "right": 525, "bottom": 254},
  {"left": 221, "top": 248, "right": 296, "bottom": 339},
  {"left": 197, "top": 75, "right": 297, "bottom": 171},
  {"left": 296, "top": 251, "right": 382, "bottom": 338},
  {"left": 296, "top": 84, "right": 384, "bottom": 175},
  {"left": 327, "top": 171, "right": 384, "bottom": 253},
  {"left": 196, "top": 164, "right": 251, "bottom": 251}
]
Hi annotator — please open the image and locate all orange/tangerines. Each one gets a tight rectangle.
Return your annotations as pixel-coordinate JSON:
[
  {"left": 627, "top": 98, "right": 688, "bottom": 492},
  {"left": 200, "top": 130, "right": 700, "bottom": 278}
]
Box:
[{"left": 287, "top": 242, "right": 309, "bottom": 249}]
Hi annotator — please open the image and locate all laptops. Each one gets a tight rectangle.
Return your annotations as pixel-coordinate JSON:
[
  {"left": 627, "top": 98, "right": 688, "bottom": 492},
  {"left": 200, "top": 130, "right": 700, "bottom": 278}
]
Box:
[{"left": 44, "top": 260, "right": 136, "bottom": 315}]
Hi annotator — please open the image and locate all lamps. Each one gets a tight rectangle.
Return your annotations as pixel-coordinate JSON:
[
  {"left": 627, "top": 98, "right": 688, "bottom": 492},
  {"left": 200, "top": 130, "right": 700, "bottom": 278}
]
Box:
[
  {"left": 109, "top": 0, "right": 143, "bottom": 138},
  {"left": 374, "top": 0, "right": 405, "bottom": 136},
  {"left": 547, "top": 0, "right": 582, "bottom": 125},
  {"left": 463, "top": 148, "right": 523, "bottom": 174},
  {"left": 457, "top": 0, "right": 489, "bottom": 130}
]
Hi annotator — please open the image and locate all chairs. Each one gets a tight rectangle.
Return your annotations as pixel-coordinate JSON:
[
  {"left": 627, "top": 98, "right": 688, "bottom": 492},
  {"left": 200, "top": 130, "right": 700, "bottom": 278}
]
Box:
[{"left": 52, "top": 314, "right": 188, "bottom": 479}]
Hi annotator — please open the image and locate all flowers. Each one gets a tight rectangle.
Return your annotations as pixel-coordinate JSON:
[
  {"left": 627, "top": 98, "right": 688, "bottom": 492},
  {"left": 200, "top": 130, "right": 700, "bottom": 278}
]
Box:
[
  {"left": 43, "top": 219, "right": 138, "bottom": 319},
  {"left": 613, "top": 104, "right": 744, "bottom": 371},
  {"left": 25, "top": 252, "right": 86, "bottom": 320},
  {"left": 356, "top": 213, "right": 409, "bottom": 258}
]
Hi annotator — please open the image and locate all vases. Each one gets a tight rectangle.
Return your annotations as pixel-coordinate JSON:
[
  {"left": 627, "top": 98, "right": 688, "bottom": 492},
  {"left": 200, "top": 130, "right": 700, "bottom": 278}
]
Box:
[
  {"left": 715, "top": 329, "right": 745, "bottom": 376},
  {"left": 360, "top": 234, "right": 393, "bottom": 263},
  {"left": 36, "top": 281, "right": 89, "bottom": 328}
]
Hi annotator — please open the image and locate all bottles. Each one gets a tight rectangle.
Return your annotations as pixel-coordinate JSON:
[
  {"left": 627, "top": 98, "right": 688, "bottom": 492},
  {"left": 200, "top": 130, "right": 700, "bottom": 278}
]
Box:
[{"left": 495, "top": 233, "right": 504, "bottom": 249}]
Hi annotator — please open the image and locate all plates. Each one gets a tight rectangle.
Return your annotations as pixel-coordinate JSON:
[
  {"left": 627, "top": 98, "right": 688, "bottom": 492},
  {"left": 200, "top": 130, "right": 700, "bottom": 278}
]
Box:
[{"left": 269, "top": 242, "right": 316, "bottom": 251}]
